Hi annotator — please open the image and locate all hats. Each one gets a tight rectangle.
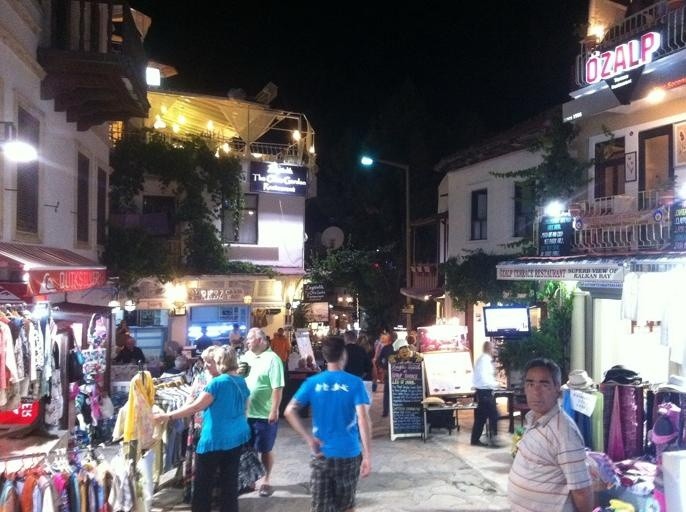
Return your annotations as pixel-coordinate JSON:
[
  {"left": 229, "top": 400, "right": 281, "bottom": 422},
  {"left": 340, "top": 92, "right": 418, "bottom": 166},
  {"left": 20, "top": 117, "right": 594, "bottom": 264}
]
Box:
[
  {"left": 603, "top": 365, "right": 644, "bottom": 385},
  {"left": 393, "top": 338, "right": 409, "bottom": 352},
  {"left": 202, "top": 326, "right": 206, "bottom": 328},
  {"left": 652, "top": 416, "right": 677, "bottom": 445},
  {"left": 233, "top": 322, "right": 241, "bottom": 326},
  {"left": 560, "top": 369, "right": 595, "bottom": 392},
  {"left": 658, "top": 374, "right": 684, "bottom": 393},
  {"left": 78, "top": 373, "right": 96, "bottom": 385},
  {"left": 95, "top": 373, "right": 105, "bottom": 382}
]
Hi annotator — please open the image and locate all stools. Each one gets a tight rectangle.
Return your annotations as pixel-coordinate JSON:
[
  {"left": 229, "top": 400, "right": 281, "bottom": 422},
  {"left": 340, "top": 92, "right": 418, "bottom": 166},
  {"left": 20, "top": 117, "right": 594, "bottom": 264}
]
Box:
[{"left": 511, "top": 403, "right": 531, "bottom": 427}]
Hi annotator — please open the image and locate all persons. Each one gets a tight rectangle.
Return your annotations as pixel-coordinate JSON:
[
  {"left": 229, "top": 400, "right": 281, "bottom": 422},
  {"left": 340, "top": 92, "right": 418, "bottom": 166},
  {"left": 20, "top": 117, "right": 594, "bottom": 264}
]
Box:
[
  {"left": 197, "top": 326, "right": 212, "bottom": 350},
  {"left": 271, "top": 328, "right": 292, "bottom": 373},
  {"left": 229, "top": 324, "right": 243, "bottom": 346},
  {"left": 152, "top": 344, "right": 252, "bottom": 512},
  {"left": 376, "top": 333, "right": 395, "bottom": 418},
  {"left": 418, "top": 329, "right": 438, "bottom": 351},
  {"left": 180, "top": 345, "right": 220, "bottom": 445},
  {"left": 114, "top": 338, "right": 146, "bottom": 364},
  {"left": 74, "top": 380, "right": 98, "bottom": 436},
  {"left": 343, "top": 331, "right": 367, "bottom": 377},
  {"left": 411, "top": 328, "right": 417, "bottom": 336},
  {"left": 237, "top": 327, "right": 285, "bottom": 498},
  {"left": 405, "top": 336, "right": 417, "bottom": 351},
  {"left": 470, "top": 341, "right": 503, "bottom": 448},
  {"left": 283, "top": 337, "right": 372, "bottom": 512},
  {"left": 507, "top": 358, "right": 595, "bottom": 512}
]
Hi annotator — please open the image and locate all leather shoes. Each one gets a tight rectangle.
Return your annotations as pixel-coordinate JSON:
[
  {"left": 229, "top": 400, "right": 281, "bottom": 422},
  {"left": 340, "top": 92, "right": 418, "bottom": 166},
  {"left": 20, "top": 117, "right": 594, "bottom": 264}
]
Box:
[
  {"left": 381, "top": 414, "right": 388, "bottom": 417},
  {"left": 490, "top": 443, "right": 504, "bottom": 448},
  {"left": 471, "top": 440, "right": 486, "bottom": 448}
]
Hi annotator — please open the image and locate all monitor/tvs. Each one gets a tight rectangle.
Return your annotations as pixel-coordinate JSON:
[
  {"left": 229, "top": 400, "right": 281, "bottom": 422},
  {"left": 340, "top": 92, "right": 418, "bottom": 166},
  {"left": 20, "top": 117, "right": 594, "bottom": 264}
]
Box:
[{"left": 483, "top": 307, "right": 532, "bottom": 336}]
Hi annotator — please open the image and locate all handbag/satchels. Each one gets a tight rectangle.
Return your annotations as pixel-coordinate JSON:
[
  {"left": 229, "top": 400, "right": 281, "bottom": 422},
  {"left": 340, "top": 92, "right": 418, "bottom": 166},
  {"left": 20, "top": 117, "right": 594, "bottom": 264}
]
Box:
[
  {"left": 69, "top": 330, "right": 83, "bottom": 383},
  {"left": 224, "top": 372, "right": 247, "bottom": 401},
  {"left": 72, "top": 330, "right": 86, "bottom": 366},
  {"left": 239, "top": 451, "right": 266, "bottom": 483},
  {"left": 237, "top": 471, "right": 255, "bottom": 497}
]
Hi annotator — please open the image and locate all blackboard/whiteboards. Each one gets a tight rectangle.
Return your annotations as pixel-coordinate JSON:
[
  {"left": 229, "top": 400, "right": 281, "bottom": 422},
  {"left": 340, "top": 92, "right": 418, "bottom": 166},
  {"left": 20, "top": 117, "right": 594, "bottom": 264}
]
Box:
[{"left": 388, "top": 358, "right": 428, "bottom": 442}]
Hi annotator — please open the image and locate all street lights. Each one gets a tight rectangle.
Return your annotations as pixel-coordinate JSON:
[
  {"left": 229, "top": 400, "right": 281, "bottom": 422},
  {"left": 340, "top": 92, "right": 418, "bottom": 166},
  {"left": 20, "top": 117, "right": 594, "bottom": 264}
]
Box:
[
  {"left": 0, "top": 121, "right": 38, "bottom": 163},
  {"left": 170, "top": 301, "right": 188, "bottom": 316},
  {"left": 361, "top": 156, "right": 413, "bottom": 331}
]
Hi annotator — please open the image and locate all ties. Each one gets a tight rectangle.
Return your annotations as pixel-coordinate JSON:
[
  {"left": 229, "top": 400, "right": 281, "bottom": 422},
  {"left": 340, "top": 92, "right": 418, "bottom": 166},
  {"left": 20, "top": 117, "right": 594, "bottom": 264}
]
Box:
[{"left": 379, "top": 344, "right": 381, "bottom": 350}]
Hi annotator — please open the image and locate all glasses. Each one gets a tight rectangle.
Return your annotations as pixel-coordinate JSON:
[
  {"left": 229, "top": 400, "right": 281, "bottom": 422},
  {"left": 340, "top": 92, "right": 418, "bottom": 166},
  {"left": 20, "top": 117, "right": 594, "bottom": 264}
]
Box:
[
  {"left": 398, "top": 348, "right": 410, "bottom": 353},
  {"left": 524, "top": 382, "right": 554, "bottom": 391},
  {"left": 236, "top": 326, "right": 239, "bottom": 327}
]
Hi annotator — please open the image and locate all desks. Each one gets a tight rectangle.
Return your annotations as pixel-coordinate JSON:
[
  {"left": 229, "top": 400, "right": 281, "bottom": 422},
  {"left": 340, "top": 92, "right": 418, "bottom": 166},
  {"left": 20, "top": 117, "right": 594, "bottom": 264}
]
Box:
[{"left": 420, "top": 404, "right": 492, "bottom": 444}]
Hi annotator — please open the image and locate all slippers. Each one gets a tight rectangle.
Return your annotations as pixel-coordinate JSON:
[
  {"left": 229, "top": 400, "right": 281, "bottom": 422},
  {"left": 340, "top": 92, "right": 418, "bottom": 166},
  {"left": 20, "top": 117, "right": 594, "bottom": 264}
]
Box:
[{"left": 259, "top": 485, "right": 276, "bottom": 497}]
